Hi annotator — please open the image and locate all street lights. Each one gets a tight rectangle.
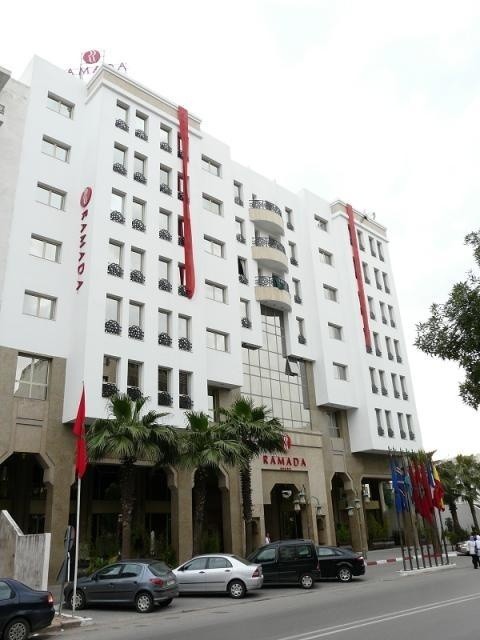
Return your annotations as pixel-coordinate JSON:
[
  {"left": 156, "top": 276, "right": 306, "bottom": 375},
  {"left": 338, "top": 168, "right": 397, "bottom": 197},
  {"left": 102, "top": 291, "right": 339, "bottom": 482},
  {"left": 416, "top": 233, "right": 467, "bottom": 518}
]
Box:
[
  {"left": 293, "top": 485, "right": 322, "bottom": 539},
  {"left": 343, "top": 485, "right": 372, "bottom": 556}
]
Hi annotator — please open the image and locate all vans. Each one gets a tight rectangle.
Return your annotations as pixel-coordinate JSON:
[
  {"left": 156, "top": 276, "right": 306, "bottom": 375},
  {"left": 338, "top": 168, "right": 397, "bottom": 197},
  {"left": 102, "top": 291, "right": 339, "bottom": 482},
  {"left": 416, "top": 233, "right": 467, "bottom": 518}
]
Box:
[
  {"left": 246, "top": 538, "right": 322, "bottom": 590},
  {"left": 62, "top": 558, "right": 179, "bottom": 614}
]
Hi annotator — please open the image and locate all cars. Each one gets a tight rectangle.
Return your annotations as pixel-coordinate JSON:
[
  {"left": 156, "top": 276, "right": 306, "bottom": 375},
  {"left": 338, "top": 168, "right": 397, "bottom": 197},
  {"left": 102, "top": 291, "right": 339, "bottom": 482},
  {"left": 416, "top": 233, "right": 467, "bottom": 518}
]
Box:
[
  {"left": 0, "top": 578, "right": 57, "bottom": 640},
  {"left": 456, "top": 540, "right": 472, "bottom": 555},
  {"left": 172, "top": 552, "right": 264, "bottom": 600},
  {"left": 315, "top": 544, "right": 367, "bottom": 584}
]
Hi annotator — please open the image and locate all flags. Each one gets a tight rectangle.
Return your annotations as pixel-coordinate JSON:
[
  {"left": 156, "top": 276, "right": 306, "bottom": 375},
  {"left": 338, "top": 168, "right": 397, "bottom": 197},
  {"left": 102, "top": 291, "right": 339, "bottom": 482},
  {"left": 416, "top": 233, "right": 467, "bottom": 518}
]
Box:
[
  {"left": 388, "top": 452, "right": 446, "bottom": 526},
  {"left": 72, "top": 388, "right": 89, "bottom": 480}
]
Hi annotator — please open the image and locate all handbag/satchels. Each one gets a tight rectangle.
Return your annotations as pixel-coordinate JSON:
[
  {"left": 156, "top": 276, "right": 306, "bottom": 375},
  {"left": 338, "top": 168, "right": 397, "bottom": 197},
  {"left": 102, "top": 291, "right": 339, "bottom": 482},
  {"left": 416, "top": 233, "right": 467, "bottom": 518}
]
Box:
[{"left": 475, "top": 542, "right": 478, "bottom": 553}]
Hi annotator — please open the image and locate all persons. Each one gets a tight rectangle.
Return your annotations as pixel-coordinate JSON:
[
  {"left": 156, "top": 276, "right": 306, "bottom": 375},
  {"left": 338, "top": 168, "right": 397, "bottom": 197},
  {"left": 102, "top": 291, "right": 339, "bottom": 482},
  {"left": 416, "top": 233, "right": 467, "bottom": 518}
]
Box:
[
  {"left": 467, "top": 531, "right": 480, "bottom": 569},
  {"left": 265, "top": 531, "right": 271, "bottom": 544}
]
